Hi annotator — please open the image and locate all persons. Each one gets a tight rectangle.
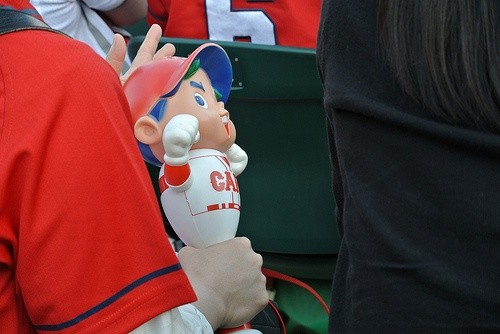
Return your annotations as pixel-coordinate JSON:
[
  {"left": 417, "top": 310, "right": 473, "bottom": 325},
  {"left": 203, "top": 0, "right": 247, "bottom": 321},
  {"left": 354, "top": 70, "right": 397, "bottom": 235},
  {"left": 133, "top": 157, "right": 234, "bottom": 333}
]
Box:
[
  {"left": 311, "top": 0, "right": 500, "bottom": 334},
  {"left": 144, "top": 0, "right": 324, "bottom": 48},
  {"left": 0, "top": 0, "right": 266, "bottom": 334},
  {"left": 32, "top": 0, "right": 139, "bottom": 86},
  {"left": 125, "top": 41, "right": 266, "bottom": 334}
]
